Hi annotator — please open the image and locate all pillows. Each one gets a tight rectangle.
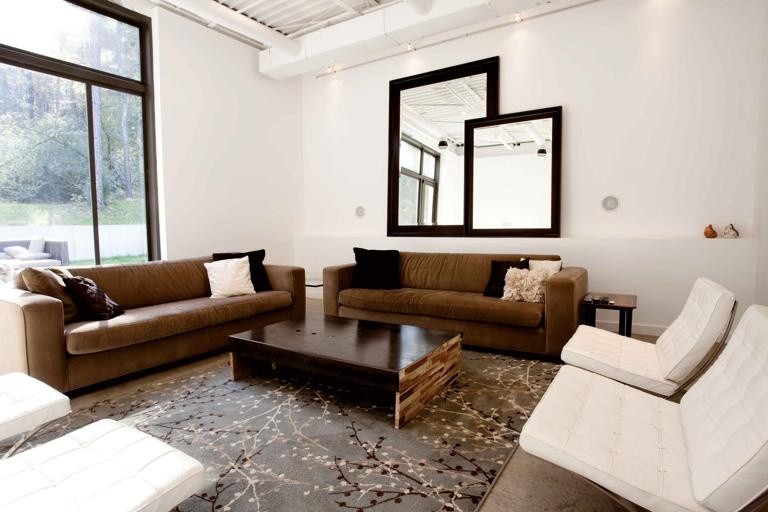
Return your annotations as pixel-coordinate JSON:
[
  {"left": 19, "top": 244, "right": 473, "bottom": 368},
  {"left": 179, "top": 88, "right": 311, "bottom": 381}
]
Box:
[
  {"left": 29, "top": 237, "right": 46, "bottom": 252},
  {"left": 204, "top": 256, "right": 256, "bottom": 299},
  {"left": 519, "top": 257, "right": 562, "bottom": 278},
  {"left": 3, "top": 245, "right": 29, "bottom": 258},
  {"left": 62, "top": 275, "right": 125, "bottom": 320},
  {"left": 482, "top": 257, "right": 530, "bottom": 298},
  {"left": 351, "top": 246, "right": 402, "bottom": 290},
  {"left": 21, "top": 266, "right": 75, "bottom": 325},
  {"left": 17, "top": 252, "right": 51, "bottom": 260},
  {"left": 212, "top": 249, "right": 274, "bottom": 293}
]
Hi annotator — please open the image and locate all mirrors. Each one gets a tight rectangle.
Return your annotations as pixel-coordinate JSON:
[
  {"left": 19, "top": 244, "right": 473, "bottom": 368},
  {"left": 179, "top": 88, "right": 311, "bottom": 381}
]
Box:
[
  {"left": 386, "top": 55, "right": 500, "bottom": 238},
  {"left": 461, "top": 106, "right": 564, "bottom": 238}
]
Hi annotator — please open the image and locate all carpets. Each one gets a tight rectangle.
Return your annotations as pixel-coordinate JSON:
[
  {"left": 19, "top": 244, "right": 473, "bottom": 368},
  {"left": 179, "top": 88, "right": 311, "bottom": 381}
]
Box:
[{"left": 0, "top": 347, "right": 563, "bottom": 510}]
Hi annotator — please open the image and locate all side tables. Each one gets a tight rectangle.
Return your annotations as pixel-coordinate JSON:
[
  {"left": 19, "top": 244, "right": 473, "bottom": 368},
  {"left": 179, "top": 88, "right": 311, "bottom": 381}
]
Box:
[{"left": 581, "top": 292, "right": 638, "bottom": 337}]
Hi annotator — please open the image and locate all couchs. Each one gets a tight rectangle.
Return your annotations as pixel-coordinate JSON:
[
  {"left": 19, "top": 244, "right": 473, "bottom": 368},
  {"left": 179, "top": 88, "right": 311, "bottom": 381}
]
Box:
[{"left": 0, "top": 240, "right": 70, "bottom": 267}]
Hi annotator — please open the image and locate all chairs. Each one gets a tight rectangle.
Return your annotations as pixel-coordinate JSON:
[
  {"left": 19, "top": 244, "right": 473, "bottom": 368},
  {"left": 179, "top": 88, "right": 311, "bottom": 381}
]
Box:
[
  {"left": 0, "top": 418, "right": 205, "bottom": 510},
  {"left": 0, "top": 371, "right": 72, "bottom": 456},
  {"left": 518, "top": 303, "right": 767, "bottom": 511},
  {"left": 558, "top": 276, "right": 737, "bottom": 397}
]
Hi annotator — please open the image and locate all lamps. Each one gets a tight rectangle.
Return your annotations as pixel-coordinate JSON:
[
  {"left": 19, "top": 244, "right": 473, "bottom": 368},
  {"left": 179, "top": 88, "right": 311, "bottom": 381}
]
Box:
[
  {"left": 531, "top": 133, "right": 554, "bottom": 161},
  {"left": 439, "top": 132, "right": 461, "bottom": 153}
]
